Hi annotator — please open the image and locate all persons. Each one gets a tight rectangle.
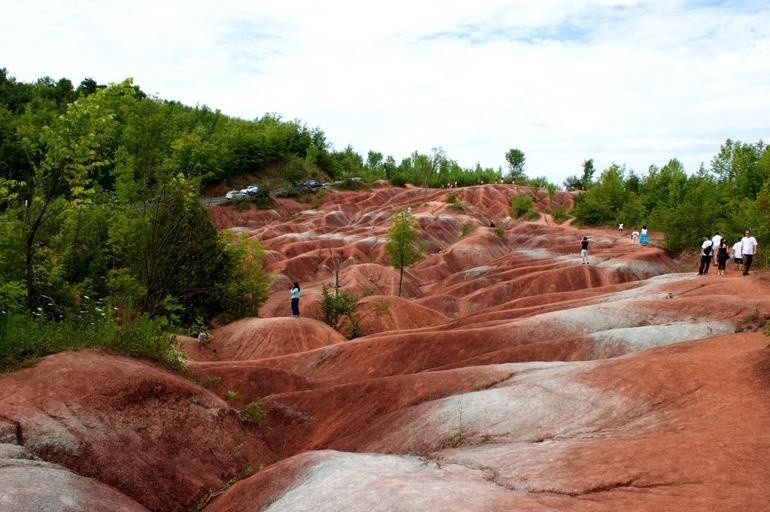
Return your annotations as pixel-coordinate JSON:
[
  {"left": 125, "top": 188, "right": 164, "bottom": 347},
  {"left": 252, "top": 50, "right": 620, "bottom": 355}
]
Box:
[
  {"left": 512, "top": 176, "right": 516, "bottom": 184},
  {"left": 696, "top": 229, "right": 758, "bottom": 275},
  {"left": 407, "top": 205, "right": 413, "bottom": 217},
  {"left": 290, "top": 280, "right": 301, "bottom": 319},
  {"left": 618, "top": 221, "right": 624, "bottom": 233},
  {"left": 500, "top": 177, "right": 504, "bottom": 185},
  {"left": 489, "top": 220, "right": 495, "bottom": 227},
  {"left": 639, "top": 226, "right": 650, "bottom": 246},
  {"left": 632, "top": 227, "right": 639, "bottom": 248},
  {"left": 580, "top": 237, "right": 590, "bottom": 265},
  {"left": 197, "top": 331, "right": 215, "bottom": 350},
  {"left": 447, "top": 180, "right": 458, "bottom": 189}
]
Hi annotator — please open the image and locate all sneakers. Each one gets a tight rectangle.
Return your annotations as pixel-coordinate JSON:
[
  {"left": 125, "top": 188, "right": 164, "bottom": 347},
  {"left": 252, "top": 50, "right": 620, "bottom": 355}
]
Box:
[
  {"left": 736, "top": 267, "right": 750, "bottom": 276},
  {"left": 697, "top": 263, "right": 725, "bottom": 275},
  {"left": 582, "top": 261, "right": 590, "bottom": 265}
]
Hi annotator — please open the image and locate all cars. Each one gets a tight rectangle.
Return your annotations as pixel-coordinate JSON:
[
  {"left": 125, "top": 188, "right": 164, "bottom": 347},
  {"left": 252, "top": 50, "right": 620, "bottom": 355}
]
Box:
[
  {"left": 303, "top": 180, "right": 324, "bottom": 188},
  {"left": 240, "top": 185, "right": 258, "bottom": 195}
]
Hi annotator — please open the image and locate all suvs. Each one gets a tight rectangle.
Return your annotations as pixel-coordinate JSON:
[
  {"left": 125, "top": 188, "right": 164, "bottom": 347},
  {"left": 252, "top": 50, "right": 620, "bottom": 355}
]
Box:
[{"left": 224, "top": 191, "right": 246, "bottom": 200}]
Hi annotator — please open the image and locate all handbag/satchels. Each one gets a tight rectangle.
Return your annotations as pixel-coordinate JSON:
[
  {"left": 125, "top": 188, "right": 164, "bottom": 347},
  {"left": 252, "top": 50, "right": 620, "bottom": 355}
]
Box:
[{"left": 703, "top": 246, "right": 713, "bottom": 256}]
